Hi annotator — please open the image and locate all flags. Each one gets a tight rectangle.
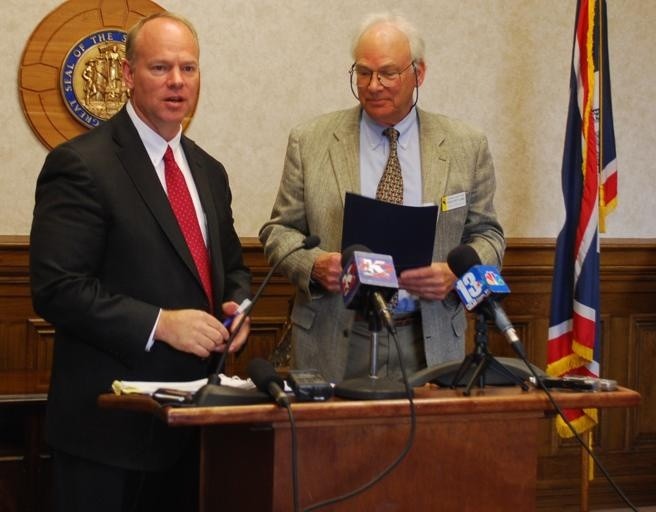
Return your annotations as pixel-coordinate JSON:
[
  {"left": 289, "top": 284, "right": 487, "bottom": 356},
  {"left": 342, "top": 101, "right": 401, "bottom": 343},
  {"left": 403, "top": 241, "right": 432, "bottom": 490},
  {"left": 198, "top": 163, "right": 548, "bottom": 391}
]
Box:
[{"left": 546, "top": 0, "right": 619, "bottom": 438}]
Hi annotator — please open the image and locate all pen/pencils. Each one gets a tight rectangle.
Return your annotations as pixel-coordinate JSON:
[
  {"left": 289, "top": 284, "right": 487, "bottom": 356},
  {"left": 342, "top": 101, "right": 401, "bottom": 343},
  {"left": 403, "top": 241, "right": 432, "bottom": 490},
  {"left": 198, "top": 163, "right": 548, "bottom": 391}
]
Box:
[{"left": 223, "top": 294, "right": 254, "bottom": 327}]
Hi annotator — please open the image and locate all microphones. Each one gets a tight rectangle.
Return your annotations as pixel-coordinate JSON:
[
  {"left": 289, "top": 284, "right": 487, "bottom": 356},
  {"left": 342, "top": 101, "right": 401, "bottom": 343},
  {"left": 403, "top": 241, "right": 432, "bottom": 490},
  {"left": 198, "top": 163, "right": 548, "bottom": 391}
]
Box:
[
  {"left": 447, "top": 244, "right": 527, "bottom": 361},
  {"left": 338, "top": 243, "right": 400, "bottom": 336},
  {"left": 246, "top": 359, "right": 290, "bottom": 406},
  {"left": 193, "top": 234, "right": 321, "bottom": 407}
]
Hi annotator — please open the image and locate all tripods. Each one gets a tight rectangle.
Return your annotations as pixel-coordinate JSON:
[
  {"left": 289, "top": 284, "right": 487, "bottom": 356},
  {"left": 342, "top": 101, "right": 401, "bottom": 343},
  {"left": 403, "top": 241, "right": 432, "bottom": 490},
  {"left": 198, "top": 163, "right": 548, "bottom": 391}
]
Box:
[{"left": 451, "top": 317, "right": 529, "bottom": 396}]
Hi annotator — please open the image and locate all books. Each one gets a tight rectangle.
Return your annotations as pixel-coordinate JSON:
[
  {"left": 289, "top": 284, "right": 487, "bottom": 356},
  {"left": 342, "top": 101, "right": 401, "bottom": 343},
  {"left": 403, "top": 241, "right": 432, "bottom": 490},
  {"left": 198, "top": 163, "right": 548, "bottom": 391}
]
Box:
[{"left": 339, "top": 189, "right": 440, "bottom": 277}]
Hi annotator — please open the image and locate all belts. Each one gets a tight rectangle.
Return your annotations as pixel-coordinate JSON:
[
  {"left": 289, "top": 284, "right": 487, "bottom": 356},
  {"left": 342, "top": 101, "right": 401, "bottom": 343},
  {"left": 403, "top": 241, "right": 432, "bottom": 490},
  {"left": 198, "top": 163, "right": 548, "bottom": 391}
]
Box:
[{"left": 354, "top": 313, "right": 416, "bottom": 327}]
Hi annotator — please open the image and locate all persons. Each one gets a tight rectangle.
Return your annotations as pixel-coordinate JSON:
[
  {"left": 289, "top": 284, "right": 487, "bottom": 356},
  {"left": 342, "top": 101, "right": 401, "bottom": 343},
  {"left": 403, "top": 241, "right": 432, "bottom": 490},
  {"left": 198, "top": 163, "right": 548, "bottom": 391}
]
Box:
[
  {"left": 26, "top": 10, "right": 255, "bottom": 512},
  {"left": 259, "top": 8, "right": 509, "bottom": 387}
]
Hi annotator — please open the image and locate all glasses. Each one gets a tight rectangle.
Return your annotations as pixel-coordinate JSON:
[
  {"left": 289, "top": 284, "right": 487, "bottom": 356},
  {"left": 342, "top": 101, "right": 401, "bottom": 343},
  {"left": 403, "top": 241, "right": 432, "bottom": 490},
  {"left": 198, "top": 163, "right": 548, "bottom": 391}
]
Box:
[{"left": 347, "top": 61, "right": 415, "bottom": 88}]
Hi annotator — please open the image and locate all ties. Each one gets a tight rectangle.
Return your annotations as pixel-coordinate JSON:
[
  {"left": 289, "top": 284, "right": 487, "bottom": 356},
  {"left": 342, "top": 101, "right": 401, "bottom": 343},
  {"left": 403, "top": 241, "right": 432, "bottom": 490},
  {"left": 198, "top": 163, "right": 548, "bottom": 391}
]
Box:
[
  {"left": 375, "top": 128, "right": 404, "bottom": 206},
  {"left": 163, "top": 144, "right": 215, "bottom": 316}
]
